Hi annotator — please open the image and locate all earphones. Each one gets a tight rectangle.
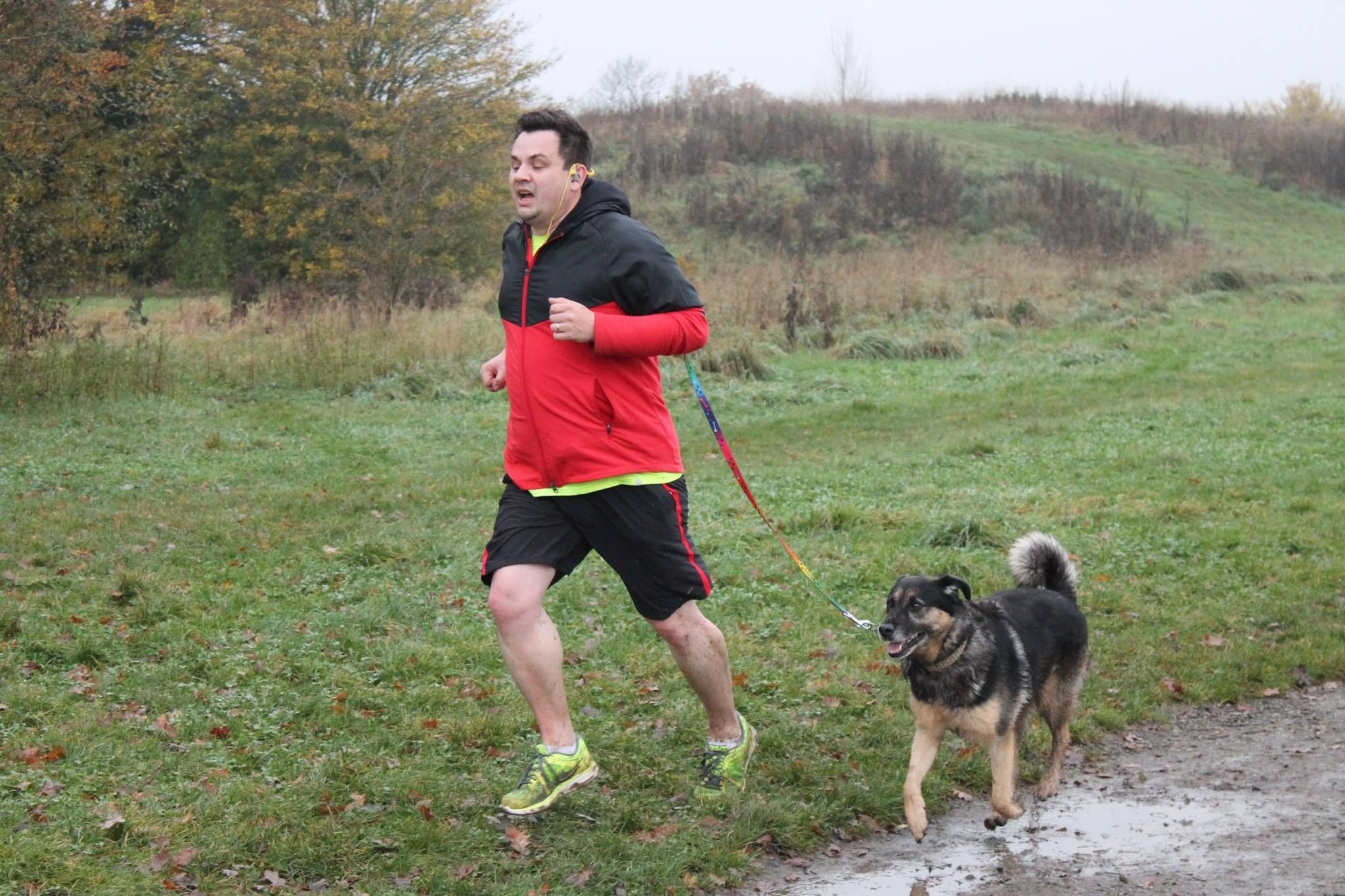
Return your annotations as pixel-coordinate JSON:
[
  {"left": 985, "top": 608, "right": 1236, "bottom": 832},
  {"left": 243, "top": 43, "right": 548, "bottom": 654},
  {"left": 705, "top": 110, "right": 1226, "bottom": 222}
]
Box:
[{"left": 570, "top": 164, "right": 581, "bottom": 182}]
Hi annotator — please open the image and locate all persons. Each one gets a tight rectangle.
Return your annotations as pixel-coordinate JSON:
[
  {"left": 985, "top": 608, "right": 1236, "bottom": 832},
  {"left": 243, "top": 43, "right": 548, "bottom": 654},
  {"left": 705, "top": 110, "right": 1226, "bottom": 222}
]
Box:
[{"left": 479, "top": 103, "right": 764, "bottom": 817}]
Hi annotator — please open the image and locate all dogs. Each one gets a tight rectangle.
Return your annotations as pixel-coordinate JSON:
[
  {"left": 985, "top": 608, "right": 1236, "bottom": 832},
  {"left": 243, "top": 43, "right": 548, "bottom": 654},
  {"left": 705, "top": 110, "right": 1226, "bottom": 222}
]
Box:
[{"left": 876, "top": 530, "right": 1089, "bottom": 844}]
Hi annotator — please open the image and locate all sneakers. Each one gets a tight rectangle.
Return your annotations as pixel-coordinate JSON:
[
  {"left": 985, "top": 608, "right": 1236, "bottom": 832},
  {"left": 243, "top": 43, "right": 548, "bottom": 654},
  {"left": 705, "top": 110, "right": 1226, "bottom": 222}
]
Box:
[
  {"left": 498, "top": 732, "right": 599, "bottom": 816},
  {"left": 692, "top": 710, "right": 757, "bottom": 799}
]
